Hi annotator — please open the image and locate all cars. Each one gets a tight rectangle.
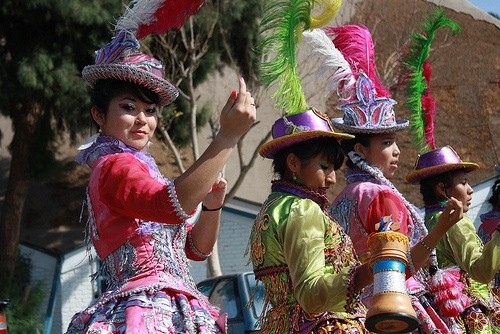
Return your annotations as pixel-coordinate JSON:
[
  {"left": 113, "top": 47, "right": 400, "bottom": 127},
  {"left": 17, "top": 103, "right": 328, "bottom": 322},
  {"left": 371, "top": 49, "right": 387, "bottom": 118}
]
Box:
[{"left": 195, "top": 272, "right": 276, "bottom": 334}]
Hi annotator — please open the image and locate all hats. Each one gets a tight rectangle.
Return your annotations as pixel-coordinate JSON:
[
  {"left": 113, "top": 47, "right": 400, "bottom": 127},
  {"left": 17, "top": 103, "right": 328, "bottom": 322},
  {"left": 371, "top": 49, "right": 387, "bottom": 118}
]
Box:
[
  {"left": 323, "top": 23, "right": 410, "bottom": 134},
  {"left": 82, "top": 0, "right": 205, "bottom": 107},
  {"left": 253, "top": 0, "right": 355, "bottom": 159},
  {"left": 393, "top": 10, "right": 480, "bottom": 183}
]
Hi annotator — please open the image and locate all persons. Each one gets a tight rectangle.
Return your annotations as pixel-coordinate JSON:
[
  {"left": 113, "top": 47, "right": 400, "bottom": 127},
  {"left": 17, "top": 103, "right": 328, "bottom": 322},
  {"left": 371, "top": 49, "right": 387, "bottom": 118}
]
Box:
[
  {"left": 243, "top": 106, "right": 373, "bottom": 334},
  {"left": 329, "top": 96, "right": 464, "bottom": 334},
  {"left": 65, "top": 35, "right": 256, "bottom": 334},
  {"left": 406, "top": 145, "right": 500, "bottom": 334}
]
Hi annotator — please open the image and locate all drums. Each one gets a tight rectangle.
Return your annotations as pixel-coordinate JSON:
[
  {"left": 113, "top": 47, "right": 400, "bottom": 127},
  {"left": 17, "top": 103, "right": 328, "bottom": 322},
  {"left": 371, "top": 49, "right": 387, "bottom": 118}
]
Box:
[{"left": 367, "top": 232, "right": 420, "bottom": 334}]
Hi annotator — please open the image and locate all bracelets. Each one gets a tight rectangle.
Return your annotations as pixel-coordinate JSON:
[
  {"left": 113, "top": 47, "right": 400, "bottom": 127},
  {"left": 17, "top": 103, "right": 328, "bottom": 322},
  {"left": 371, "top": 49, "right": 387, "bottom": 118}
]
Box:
[
  {"left": 420, "top": 240, "right": 432, "bottom": 252},
  {"left": 202, "top": 202, "right": 223, "bottom": 211}
]
